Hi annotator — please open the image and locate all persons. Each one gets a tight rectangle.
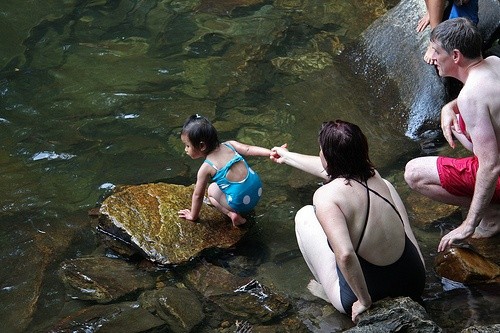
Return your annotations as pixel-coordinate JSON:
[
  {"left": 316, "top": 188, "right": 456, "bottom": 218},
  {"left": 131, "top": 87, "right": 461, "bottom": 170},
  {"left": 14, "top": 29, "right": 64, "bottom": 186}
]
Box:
[
  {"left": 269, "top": 118, "right": 428, "bottom": 324},
  {"left": 177, "top": 112, "right": 289, "bottom": 227},
  {"left": 403, "top": 17, "right": 500, "bottom": 253},
  {"left": 414, "top": 0, "right": 479, "bottom": 103}
]
